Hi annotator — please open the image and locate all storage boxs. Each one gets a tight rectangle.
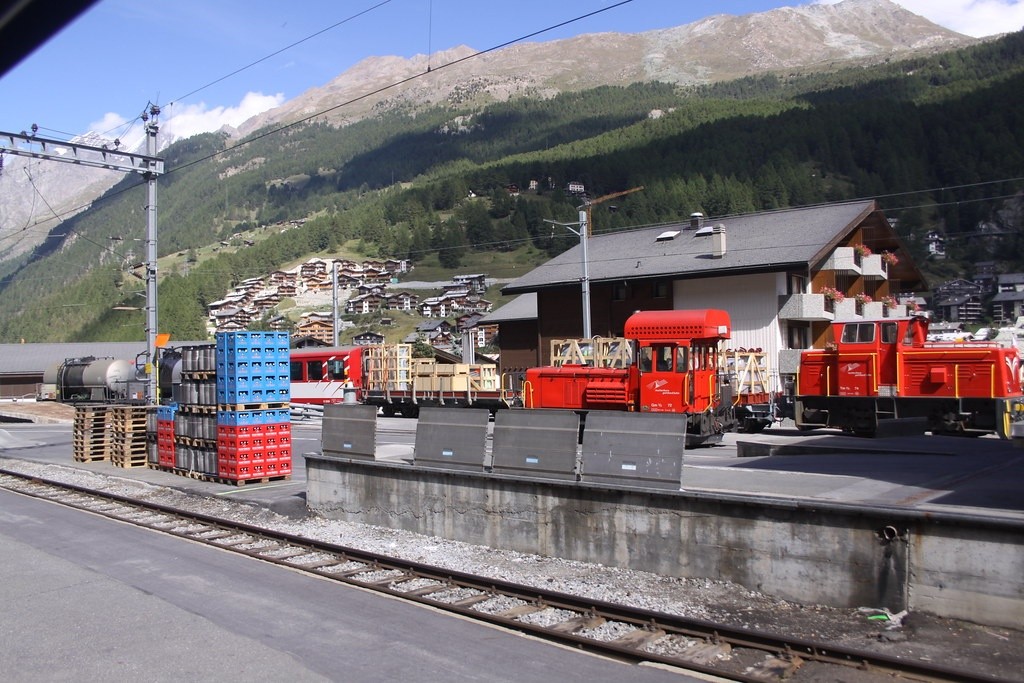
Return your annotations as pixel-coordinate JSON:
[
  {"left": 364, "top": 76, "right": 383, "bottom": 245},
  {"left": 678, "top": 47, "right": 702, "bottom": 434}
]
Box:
[{"left": 216, "top": 330, "right": 293, "bottom": 480}]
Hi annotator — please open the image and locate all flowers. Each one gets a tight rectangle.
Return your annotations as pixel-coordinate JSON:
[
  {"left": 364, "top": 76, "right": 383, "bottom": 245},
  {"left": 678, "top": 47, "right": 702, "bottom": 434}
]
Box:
[
  {"left": 882, "top": 295, "right": 897, "bottom": 309},
  {"left": 854, "top": 244, "right": 873, "bottom": 257},
  {"left": 855, "top": 292, "right": 872, "bottom": 305},
  {"left": 820, "top": 284, "right": 846, "bottom": 302},
  {"left": 905, "top": 299, "right": 921, "bottom": 314},
  {"left": 881, "top": 250, "right": 898, "bottom": 267}
]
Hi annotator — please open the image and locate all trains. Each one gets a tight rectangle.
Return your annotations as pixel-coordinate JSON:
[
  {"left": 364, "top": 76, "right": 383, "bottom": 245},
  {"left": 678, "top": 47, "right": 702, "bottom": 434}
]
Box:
[
  {"left": 35, "top": 346, "right": 182, "bottom": 405},
  {"left": 502, "top": 310, "right": 738, "bottom": 448},
  {"left": 290, "top": 315, "right": 1024, "bottom": 440}
]
